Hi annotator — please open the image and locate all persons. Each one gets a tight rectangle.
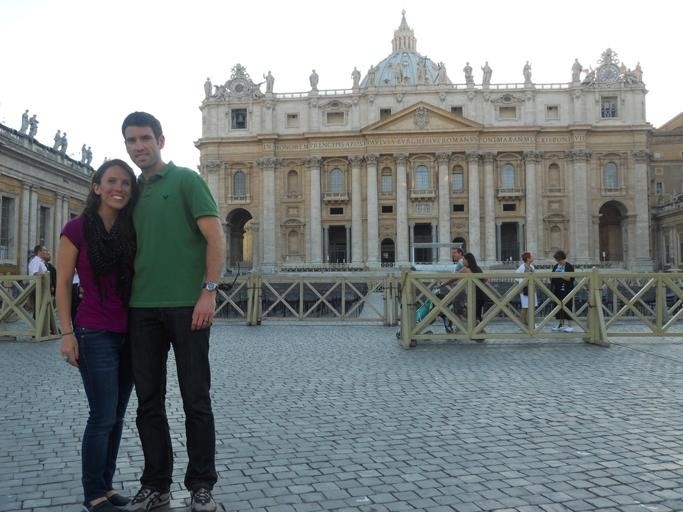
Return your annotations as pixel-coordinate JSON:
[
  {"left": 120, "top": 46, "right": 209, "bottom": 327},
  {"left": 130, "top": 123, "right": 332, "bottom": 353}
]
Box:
[
  {"left": 351, "top": 66, "right": 361, "bottom": 88},
  {"left": 309, "top": 68, "right": 319, "bottom": 92},
  {"left": 67, "top": 263, "right": 80, "bottom": 324},
  {"left": 548, "top": 249, "right": 577, "bottom": 331},
  {"left": 434, "top": 247, "right": 465, "bottom": 336},
  {"left": 514, "top": 252, "right": 537, "bottom": 328},
  {"left": 366, "top": 64, "right": 376, "bottom": 84},
  {"left": 523, "top": 60, "right": 532, "bottom": 82},
  {"left": 467, "top": 75, "right": 474, "bottom": 84},
  {"left": 480, "top": 60, "right": 492, "bottom": 85},
  {"left": 571, "top": 57, "right": 583, "bottom": 82},
  {"left": 441, "top": 254, "right": 485, "bottom": 339},
  {"left": 203, "top": 76, "right": 212, "bottom": 98},
  {"left": 76, "top": 117, "right": 227, "bottom": 511},
  {"left": 43, "top": 251, "right": 58, "bottom": 336},
  {"left": 463, "top": 61, "right": 472, "bottom": 82},
  {"left": 27, "top": 244, "right": 56, "bottom": 338},
  {"left": 416, "top": 56, "right": 427, "bottom": 81},
  {"left": 263, "top": 70, "right": 275, "bottom": 93},
  {"left": 393, "top": 62, "right": 404, "bottom": 83},
  {"left": 620, "top": 61, "right": 643, "bottom": 85},
  {"left": 435, "top": 61, "right": 446, "bottom": 82},
  {"left": 53, "top": 160, "right": 139, "bottom": 511}
]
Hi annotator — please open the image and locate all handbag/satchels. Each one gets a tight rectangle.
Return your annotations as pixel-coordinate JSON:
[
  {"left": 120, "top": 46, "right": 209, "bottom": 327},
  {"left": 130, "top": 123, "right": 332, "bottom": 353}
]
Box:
[{"left": 523, "top": 284, "right": 529, "bottom": 296}]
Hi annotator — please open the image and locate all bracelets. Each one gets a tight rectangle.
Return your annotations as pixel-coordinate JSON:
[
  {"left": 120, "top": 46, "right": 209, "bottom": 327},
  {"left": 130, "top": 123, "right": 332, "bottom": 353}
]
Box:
[
  {"left": 438, "top": 281, "right": 444, "bottom": 288},
  {"left": 60, "top": 331, "right": 74, "bottom": 337}
]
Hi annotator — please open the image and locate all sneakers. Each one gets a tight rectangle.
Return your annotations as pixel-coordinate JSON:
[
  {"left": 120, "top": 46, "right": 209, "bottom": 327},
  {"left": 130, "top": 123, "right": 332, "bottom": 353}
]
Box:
[
  {"left": 552, "top": 323, "right": 576, "bottom": 332},
  {"left": 190, "top": 484, "right": 216, "bottom": 512},
  {"left": 81, "top": 489, "right": 130, "bottom": 512},
  {"left": 123, "top": 485, "right": 170, "bottom": 512}
]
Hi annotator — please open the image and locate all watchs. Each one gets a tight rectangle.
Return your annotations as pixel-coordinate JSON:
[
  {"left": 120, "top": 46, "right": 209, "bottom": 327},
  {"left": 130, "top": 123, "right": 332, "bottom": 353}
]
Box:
[{"left": 198, "top": 280, "right": 219, "bottom": 292}]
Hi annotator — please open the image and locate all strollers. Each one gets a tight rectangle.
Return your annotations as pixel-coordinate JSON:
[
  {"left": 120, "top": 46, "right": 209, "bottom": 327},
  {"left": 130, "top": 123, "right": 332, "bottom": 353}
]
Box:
[{"left": 394, "top": 283, "right": 461, "bottom": 348}]
